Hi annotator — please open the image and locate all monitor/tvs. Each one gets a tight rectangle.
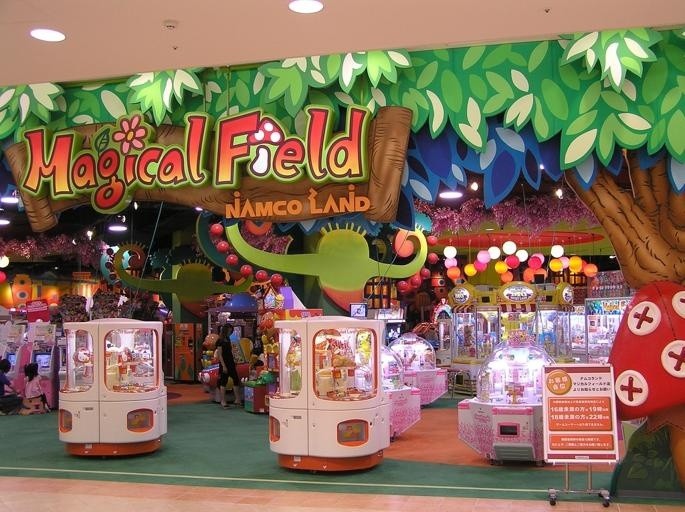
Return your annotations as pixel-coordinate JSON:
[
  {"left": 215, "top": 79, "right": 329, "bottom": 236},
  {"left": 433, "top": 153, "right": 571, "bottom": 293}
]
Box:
[
  {"left": 350, "top": 303, "right": 368, "bottom": 319},
  {"left": 6, "top": 353, "right": 17, "bottom": 365},
  {"left": 34, "top": 353, "right": 51, "bottom": 368}
]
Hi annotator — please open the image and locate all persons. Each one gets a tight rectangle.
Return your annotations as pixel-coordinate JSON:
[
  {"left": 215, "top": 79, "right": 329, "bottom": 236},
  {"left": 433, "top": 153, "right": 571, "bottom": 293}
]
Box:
[
  {"left": 23, "top": 362, "right": 51, "bottom": 413},
  {"left": 0, "top": 358, "right": 21, "bottom": 416},
  {"left": 215, "top": 321, "right": 243, "bottom": 409}
]
